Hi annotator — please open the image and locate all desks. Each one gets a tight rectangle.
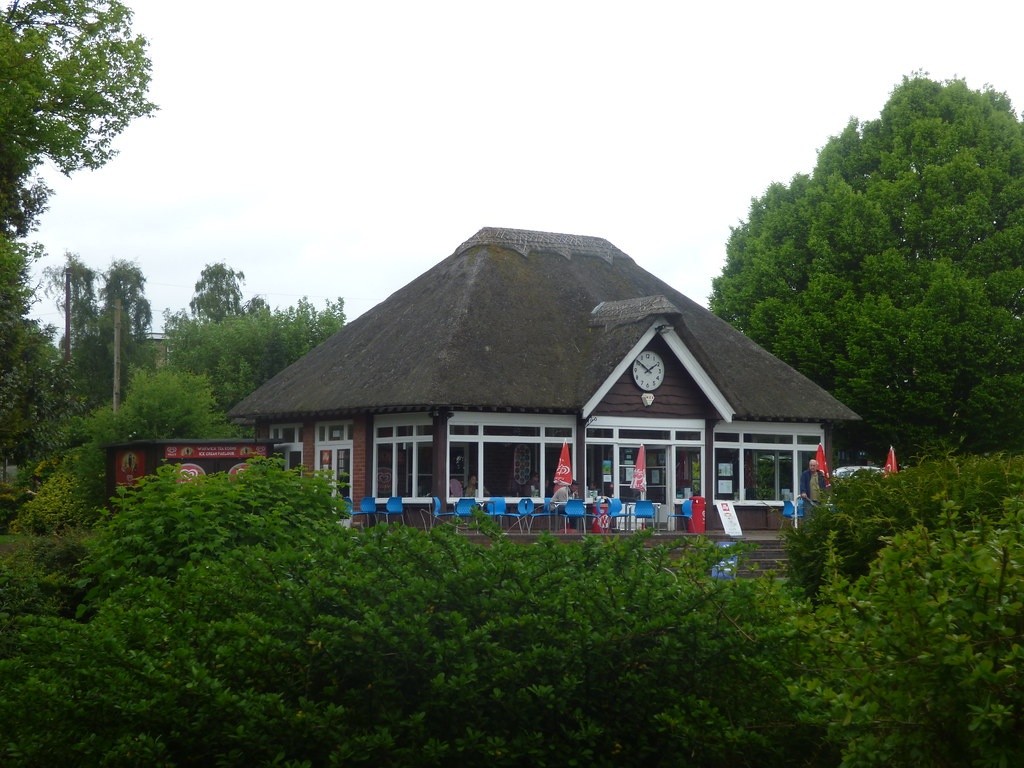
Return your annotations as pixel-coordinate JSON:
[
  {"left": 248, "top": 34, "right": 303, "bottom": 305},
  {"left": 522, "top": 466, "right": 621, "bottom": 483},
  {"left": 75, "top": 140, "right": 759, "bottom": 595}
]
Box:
[
  {"left": 455, "top": 500, "right": 496, "bottom": 530},
  {"left": 551, "top": 501, "right": 593, "bottom": 533},
  {"left": 626, "top": 501, "right": 662, "bottom": 533}
]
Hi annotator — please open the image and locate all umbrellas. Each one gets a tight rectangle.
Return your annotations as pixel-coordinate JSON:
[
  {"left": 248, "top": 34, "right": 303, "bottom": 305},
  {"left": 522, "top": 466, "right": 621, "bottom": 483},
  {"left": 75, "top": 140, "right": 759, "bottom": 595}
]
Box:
[
  {"left": 553, "top": 438, "right": 572, "bottom": 502},
  {"left": 882, "top": 446, "right": 898, "bottom": 477},
  {"left": 629, "top": 441, "right": 647, "bottom": 500},
  {"left": 814, "top": 441, "right": 830, "bottom": 488}
]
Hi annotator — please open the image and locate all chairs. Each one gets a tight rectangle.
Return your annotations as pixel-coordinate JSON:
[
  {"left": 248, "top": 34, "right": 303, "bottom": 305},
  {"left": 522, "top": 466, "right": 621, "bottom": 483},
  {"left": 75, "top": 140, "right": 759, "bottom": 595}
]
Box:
[
  {"left": 352, "top": 497, "right": 378, "bottom": 529},
  {"left": 524, "top": 498, "right": 551, "bottom": 533},
  {"left": 501, "top": 499, "right": 528, "bottom": 534},
  {"left": 666, "top": 499, "right": 695, "bottom": 532},
  {"left": 715, "top": 542, "right": 738, "bottom": 580},
  {"left": 376, "top": 496, "right": 404, "bottom": 529},
  {"left": 342, "top": 497, "right": 368, "bottom": 529},
  {"left": 429, "top": 497, "right": 453, "bottom": 527},
  {"left": 635, "top": 500, "right": 656, "bottom": 532},
  {"left": 580, "top": 499, "right": 603, "bottom": 532},
  {"left": 560, "top": 500, "right": 586, "bottom": 534},
  {"left": 454, "top": 499, "right": 478, "bottom": 534},
  {"left": 486, "top": 497, "right": 506, "bottom": 524},
  {"left": 604, "top": 497, "right": 634, "bottom": 534},
  {"left": 784, "top": 500, "right": 805, "bottom": 530}
]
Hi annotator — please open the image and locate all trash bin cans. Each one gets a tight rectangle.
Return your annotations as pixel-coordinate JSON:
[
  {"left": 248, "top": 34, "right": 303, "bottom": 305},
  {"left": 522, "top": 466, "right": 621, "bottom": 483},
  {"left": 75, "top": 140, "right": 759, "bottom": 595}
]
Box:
[
  {"left": 689, "top": 496, "right": 706, "bottom": 533},
  {"left": 593, "top": 504, "right": 609, "bottom": 534}
]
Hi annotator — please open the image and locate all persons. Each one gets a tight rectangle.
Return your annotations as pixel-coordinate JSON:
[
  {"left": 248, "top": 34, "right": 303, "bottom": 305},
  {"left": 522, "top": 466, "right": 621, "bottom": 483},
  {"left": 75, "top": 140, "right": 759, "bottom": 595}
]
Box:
[
  {"left": 549, "top": 479, "right": 577, "bottom": 530},
  {"left": 523, "top": 473, "right": 539, "bottom": 497},
  {"left": 590, "top": 481, "right": 603, "bottom": 495},
  {"left": 800, "top": 459, "right": 827, "bottom": 522},
  {"left": 461, "top": 475, "right": 477, "bottom": 497}
]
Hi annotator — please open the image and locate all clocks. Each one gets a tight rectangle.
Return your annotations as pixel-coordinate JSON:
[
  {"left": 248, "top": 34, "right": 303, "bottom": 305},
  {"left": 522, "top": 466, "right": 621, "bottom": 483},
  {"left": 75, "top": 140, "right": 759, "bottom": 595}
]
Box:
[{"left": 632, "top": 350, "right": 665, "bottom": 391}]
